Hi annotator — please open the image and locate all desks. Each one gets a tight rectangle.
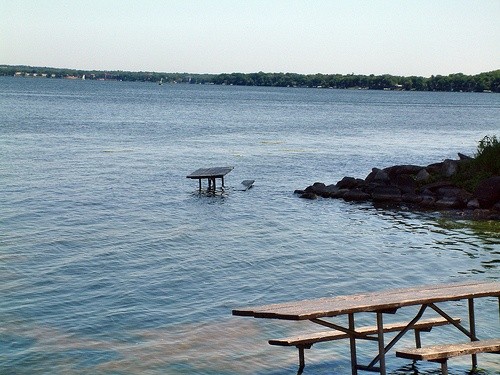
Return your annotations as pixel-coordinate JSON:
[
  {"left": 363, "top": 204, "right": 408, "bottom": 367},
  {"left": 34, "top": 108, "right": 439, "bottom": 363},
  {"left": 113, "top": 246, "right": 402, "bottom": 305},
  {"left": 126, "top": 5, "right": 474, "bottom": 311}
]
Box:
[
  {"left": 185, "top": 166, "right": 235, "bottom": 194},
  {"left": 232, "top": 280, "right": 500, "bottom": 375}
]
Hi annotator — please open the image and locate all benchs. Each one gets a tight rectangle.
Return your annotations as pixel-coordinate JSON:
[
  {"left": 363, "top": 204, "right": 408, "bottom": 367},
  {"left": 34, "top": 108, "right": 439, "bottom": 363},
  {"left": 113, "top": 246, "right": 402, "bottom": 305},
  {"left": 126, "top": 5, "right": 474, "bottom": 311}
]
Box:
[
  {"left": 267, "top": 316, "right": 460, "bottom": 368},
  {"left": 233, "top": 179, "right": 255, "bottom": 192},
  {"left": 395, "top": 338, "right": 499, "bottom": 375}
]
